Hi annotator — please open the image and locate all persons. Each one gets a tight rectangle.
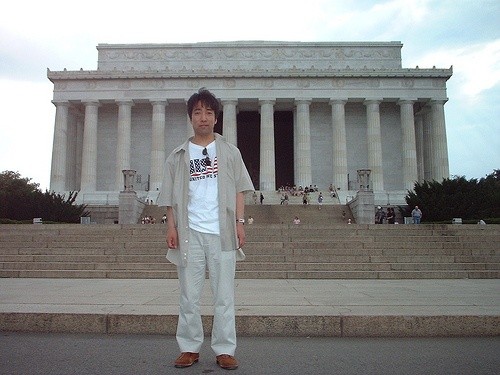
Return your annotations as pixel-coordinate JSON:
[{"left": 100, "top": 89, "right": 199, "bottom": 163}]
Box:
[
  {"left": 166, "top": 85, "right": 255, "bottom": 370},
  {"left": 141, "top": 214, "right": 166, "bottom": 224},
  {"left": 412, "top": 206, "right": 422, "bottom": 224},
  {"left": 248, "top": 216, "right": 254, "bottom": 224},
  {"left": 252, "top": 192, "right": 257, "bottom": 204},
  {"left": 375, "top": 206, "right": 395, "bottom": 224},
  {"left": 260, "top": 193, "right": 265, "bottom": 204},
  {"left": 294, "top": 217, "right": 300, "bottom": 224},
  {"left": 276, "top": 183, "right": 336, "bottom": 210}
]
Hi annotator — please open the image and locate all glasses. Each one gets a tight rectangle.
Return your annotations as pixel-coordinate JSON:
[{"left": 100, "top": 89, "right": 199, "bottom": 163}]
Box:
[{"left": 202, "top": 147, "right": 211, "bottom": 167}]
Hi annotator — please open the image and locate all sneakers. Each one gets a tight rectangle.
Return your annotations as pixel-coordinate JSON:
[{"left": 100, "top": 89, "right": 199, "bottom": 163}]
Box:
[
  {"left": 174, "top": 351, "right": 199, "bottom": 368},
  {"left": 216, "top": 354, "right": 239, "bottom": 370}
]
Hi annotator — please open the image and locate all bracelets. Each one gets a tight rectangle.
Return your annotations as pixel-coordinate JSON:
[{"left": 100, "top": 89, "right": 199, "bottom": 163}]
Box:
[{"left": 236, "top": 218, "right": 245, "bottom": 224}]
